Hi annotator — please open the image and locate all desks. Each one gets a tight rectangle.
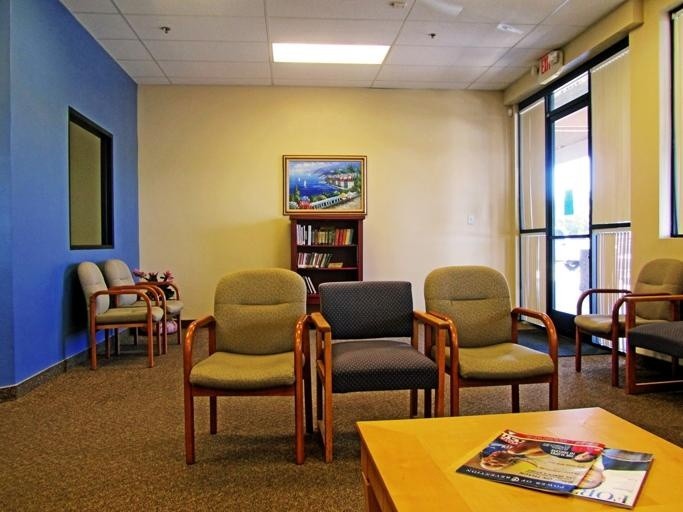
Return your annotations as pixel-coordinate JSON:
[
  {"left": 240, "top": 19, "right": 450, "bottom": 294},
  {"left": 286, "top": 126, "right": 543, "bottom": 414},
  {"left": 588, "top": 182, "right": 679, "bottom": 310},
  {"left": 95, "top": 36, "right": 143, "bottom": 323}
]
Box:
[{"left": 354, "top": 406, "right": 683, "bottom": 511}]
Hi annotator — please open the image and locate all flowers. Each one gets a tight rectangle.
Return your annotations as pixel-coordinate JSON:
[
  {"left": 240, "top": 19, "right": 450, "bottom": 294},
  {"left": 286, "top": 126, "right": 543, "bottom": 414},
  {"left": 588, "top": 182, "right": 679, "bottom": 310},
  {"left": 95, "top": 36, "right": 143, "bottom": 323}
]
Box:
[{"left": 132, "top": 268, "right": 178, "bottom": 320}]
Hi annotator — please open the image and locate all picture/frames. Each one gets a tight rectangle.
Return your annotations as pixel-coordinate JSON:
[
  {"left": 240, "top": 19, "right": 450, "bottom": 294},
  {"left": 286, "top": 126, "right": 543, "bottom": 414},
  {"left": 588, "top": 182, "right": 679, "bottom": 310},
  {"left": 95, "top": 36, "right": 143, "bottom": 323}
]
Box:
[{"left": 282, "top": 155, "right": 368, "bottom": 217}]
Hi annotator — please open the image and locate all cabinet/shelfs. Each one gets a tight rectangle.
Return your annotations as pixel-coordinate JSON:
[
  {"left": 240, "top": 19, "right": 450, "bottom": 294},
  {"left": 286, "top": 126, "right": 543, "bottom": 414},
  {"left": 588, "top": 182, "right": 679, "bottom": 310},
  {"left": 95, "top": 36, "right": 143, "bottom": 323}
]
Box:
[{"left": 289, "top": 217, "right": 365, "bottom": 331}]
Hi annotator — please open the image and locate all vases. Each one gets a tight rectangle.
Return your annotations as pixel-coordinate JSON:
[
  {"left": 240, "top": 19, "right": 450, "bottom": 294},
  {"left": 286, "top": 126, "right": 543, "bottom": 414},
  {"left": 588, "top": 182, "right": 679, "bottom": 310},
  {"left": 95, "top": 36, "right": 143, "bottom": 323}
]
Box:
[{"left": 139, "top": 320, "right": 179, "bottom": 335}]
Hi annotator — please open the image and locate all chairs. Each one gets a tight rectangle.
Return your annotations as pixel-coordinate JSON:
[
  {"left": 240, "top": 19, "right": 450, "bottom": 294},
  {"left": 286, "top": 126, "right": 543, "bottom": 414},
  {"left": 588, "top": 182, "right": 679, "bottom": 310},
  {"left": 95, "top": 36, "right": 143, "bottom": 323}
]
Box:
[
  {"left": 78, "top": 261, "right": 162, "bottom": 370},
  {"left": 183, "top": 267, "right": 309, "bottom": 466},
  {"left": 423, "top": 265, "right": 559, "bottom": 416},
  {"left": 574, "top": 258, "right": 683, "bottom": 388},
  {"left": 624, "top": 295, "right": 683, "bottom": 396},
  {"left": 310, "top": 280, "right": 448, "bottom": 464},
  {"left": 106, "top": 259, "right": 183, "bottom": 357}
]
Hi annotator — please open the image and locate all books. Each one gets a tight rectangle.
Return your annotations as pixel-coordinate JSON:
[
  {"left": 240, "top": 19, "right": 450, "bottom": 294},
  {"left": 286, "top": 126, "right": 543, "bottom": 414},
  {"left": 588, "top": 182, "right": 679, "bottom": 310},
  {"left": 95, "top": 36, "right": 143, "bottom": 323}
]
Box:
[
  {"left": 296, "top": 224, "right": 354, "bottom": 246},
  {"left": 303, "top": 276, "right": 318, "bottom": 293},
  {"left": 455, "top": 428, "right": 654, "bottom": 509},
  {"left": 298, "top": 252, "right": 343, "bottom": 268}
]
